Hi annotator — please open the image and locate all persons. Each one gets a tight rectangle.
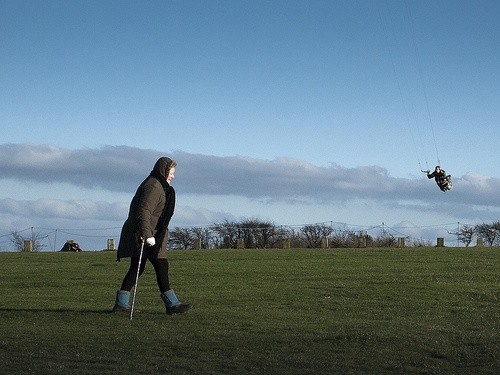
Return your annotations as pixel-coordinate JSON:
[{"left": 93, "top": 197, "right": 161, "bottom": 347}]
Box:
[
  {"left": 58, "top": 240, "right": 82, "bottom": 252},
  {"left": 427, "top": 166, "right": 452, "bottom": 192},
  {"left": 113, "top": 157, "right": 192, "bottom": 314}
]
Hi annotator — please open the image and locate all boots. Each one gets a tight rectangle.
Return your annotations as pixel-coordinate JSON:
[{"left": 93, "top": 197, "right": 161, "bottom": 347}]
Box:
[
  {"left": 160, "top": 289, "right": 193, "bottom": 315},
  {"left": 112, "top": 290, "right": 142, "bottom": 313}
]
